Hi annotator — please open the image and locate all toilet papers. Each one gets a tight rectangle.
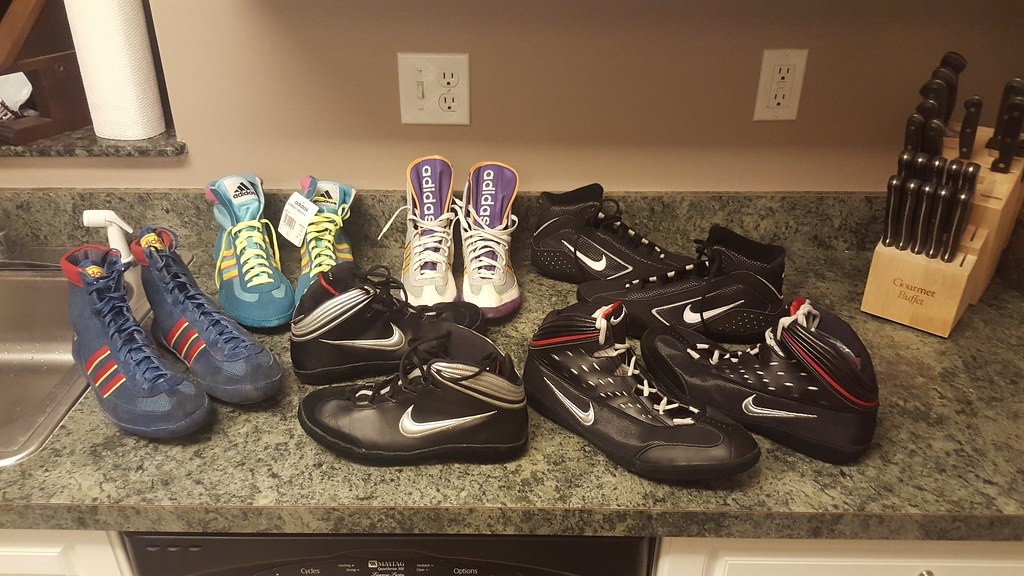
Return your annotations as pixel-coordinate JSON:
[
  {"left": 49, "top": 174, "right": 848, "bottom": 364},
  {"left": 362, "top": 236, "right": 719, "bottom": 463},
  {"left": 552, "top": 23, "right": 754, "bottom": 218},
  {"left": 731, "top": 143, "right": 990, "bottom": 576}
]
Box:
[{"left": 65, "top": 0, "right": 167, "bottom": 141}]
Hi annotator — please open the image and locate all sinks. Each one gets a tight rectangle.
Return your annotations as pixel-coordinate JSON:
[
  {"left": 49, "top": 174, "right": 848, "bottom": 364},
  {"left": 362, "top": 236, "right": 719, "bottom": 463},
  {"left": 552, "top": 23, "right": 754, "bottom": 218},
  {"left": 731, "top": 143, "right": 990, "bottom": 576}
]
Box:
[{"left": 0, "top": 246, "right": 194, "bottom": 468}]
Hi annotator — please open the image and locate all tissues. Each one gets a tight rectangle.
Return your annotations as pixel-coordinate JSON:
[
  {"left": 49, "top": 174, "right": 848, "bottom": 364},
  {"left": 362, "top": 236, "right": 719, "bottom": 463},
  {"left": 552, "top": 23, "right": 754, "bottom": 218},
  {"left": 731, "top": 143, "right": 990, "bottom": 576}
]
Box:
[{"left": 0, "top": 69, "right": 56, "bottom": 143}]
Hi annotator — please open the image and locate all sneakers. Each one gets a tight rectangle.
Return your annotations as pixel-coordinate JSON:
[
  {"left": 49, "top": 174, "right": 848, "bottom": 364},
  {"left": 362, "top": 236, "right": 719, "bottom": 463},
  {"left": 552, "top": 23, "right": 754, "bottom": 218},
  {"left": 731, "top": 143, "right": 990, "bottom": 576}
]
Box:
[
  {"left": 298, "top": 335, "right": 529, "bottom": 464},
  {"left": 377, "top": 155, "right": 460, "bottom": 312},
  {"left": 524, "top": 302, "right": 762, "bottom": 486},
  {"left": 641, "top": 303, "right": 881, "bottom": 464},
  {"left": 531, "top": 183, "right": 695, "bottom": 286},
  {"left": 60, "top": 243, "right": 210, "bottom": 440},
  {"left": 296, "top": 176, "right": 356, "bottom": 304},
  {"left": 204, "top": 176, "right": 293, "bottom": 329},
  {"left": 293, "top": 263, "right": 484, "bottom": 381},
  {"left": 455, "top": 162, "right": 521, "bottom": 325},
  {"left": 576, "top": 226, "right": 786, "bottom": 342},
  {"left": 132, "top": 228, "right": 285, "bottom": 404}
]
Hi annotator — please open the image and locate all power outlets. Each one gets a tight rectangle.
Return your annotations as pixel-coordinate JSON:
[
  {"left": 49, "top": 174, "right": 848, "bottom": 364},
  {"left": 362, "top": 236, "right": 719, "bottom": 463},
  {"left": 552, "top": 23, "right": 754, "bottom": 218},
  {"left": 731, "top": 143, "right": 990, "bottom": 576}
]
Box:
[
  {"left": 752, "top": 48, "right": 809, "bottom": 122},
  {"left": 396, "top": 51, "right": 471, "bottom": 126}
]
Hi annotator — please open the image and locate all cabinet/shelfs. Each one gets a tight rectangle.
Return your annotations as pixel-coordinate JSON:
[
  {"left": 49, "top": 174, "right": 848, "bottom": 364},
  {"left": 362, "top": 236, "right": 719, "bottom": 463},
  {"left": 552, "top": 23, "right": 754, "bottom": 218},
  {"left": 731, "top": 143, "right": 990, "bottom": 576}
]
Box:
[{"left": 651, "top": 537, "right": 1024, "bottom": 576}]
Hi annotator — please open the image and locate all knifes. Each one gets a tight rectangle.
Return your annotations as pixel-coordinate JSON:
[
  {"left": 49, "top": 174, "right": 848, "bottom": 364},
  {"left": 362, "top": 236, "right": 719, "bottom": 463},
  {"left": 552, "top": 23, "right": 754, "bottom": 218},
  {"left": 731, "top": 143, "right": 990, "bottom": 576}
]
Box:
[{"left": 882, "top": 48, "right": 1024, "bottom": 262}]
